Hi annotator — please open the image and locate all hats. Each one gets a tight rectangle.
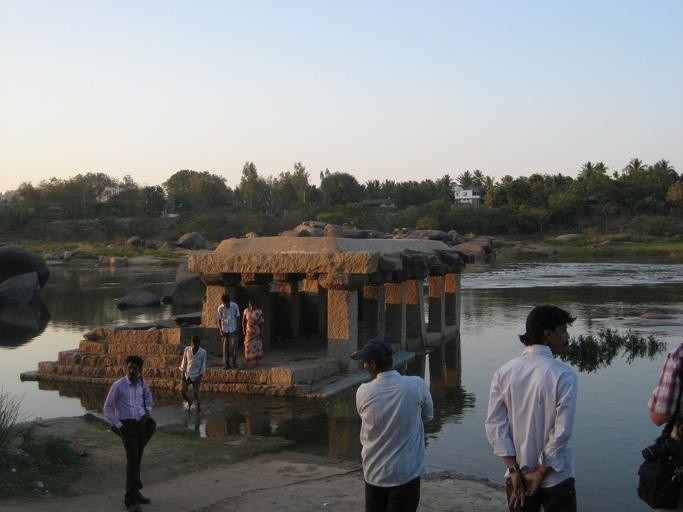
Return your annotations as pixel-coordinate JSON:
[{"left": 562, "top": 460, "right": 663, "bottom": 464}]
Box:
[{"left": 349, "top": 337, "right": 392, "bottom": 360}]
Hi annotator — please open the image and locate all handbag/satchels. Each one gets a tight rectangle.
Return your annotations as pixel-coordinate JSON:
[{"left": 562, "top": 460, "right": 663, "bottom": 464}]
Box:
[{"left": 637, "top": 434, "right": 683, "bottom": 512}]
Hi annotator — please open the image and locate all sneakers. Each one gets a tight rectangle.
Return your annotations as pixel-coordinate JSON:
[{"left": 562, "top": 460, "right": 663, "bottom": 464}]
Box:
[{"left": 127, "top": 491, "right": 151, "bottom": 512}]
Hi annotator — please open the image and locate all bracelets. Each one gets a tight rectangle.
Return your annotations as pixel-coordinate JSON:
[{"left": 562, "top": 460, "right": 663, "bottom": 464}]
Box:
[{"left": 508, "top": 464, "right": 520, "bottom": 473}]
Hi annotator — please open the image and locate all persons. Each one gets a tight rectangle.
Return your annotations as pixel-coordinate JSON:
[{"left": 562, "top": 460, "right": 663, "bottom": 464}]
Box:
[
  {"left": 178, "top": 335, "right": 207, "bottom": 411},
  {"left": 349, "top": 337, "right": 434, "bottom": 512},
  {"left": 241, "top": 298, "right": 264, "bottom": 364},
  {"left": 217, "top": 294, "right": 241, "bottom": 369},
  {"left": 103, "top": 354, "right": 154, "bottom": 512},
  {"left": 484, "top": 304, "right": 579, "bottom": 511},
  {"left": 647, "top": 341, "right": 683, "bottom": 440}
]
